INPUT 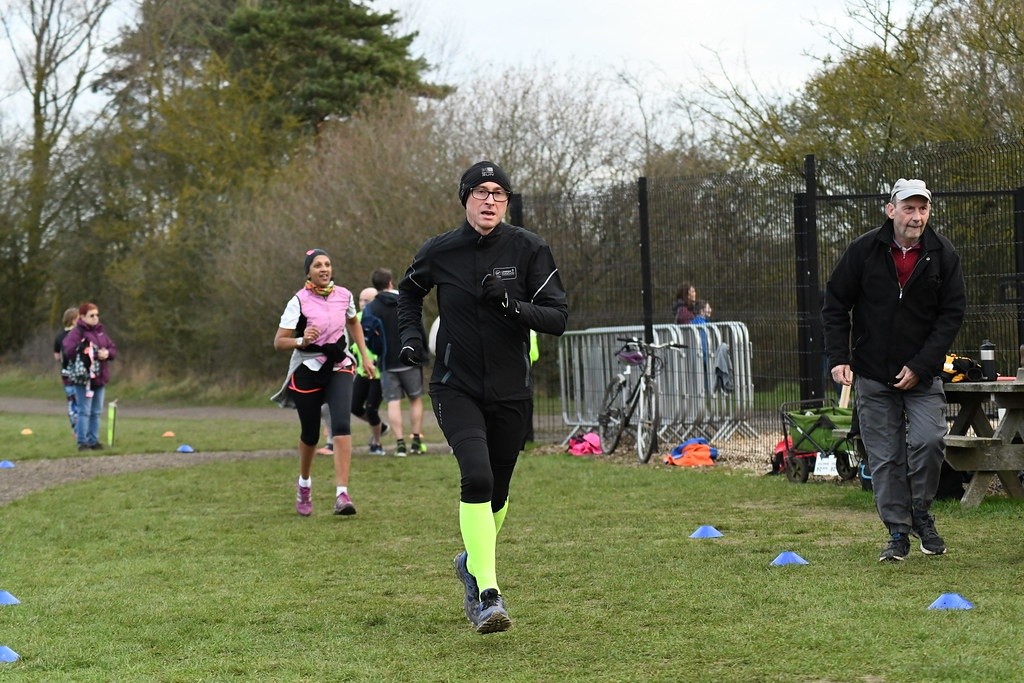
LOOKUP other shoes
[78,445,90,452]
[91,443,103,450]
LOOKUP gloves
[481,273,513,318]
[396,337,431,367]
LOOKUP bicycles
[597,336,688,463]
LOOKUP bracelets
[297,338,303,347]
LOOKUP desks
[944,378,1024,510]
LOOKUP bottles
[980,339,997,381]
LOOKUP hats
[890,177,933,203]
[458,161,512,210]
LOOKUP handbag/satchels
[358,304,387,357]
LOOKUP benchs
[833,426,1002,473]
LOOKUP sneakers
[296,482,312,517]
[368,443,385,456]
[316,443,333,455]
[454,551,481,625]
[880,533,912,561]
[324,425,329,437]
[393,445,407,457]
[409,441,427,455]
[381,422,389,436]
[476,588,511,633]
[911,514,946,555]
[333,492,356,516]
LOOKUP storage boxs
[790,407,856,450]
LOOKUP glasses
[90,314,101,317]
[468,187,511,202]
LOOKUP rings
[308,337,310,338]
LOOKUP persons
[428,315,453,455]
[312,287,391,455]
[821,179,966,562]
[271,249,377,516]
[62,303,116,450]
[53,307,79,435]
[361,268,426,456]
[530,329,539,365]
[397,162,568,634]
[674,282,711,393]
